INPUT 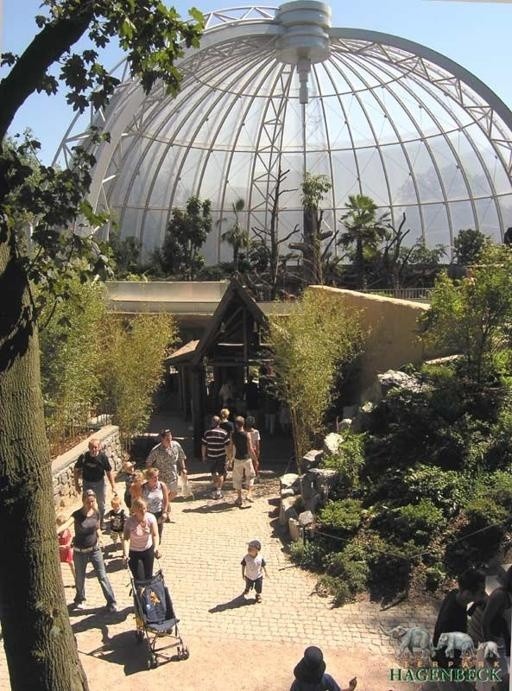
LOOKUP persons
[218,409,235,470]
[278,403,290,433]
[73,438,117,531]
[56,513,76,580]
[114,461,142,509]
[121,498,162,585]
[140,467,170,546]
[241,540,267,603]
[228,415,261,504]
[243,414,262,480]
[262,393,278,434]
[56,488,117,613]
[290,645,358,690]
[201,414,231,500]
[239,374,259,412]
[145,427,188,523]
[55,514,76,589]
[431,567,478,670]
[102,494,126,557]
[465,564,512,690]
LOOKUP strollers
[125,553,190,670]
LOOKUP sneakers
[216,493,224,498]
[256,594,262,603]
[106,602,118,612]
[246,496,254,503]
[233,496,243,505]
[210,492,217,499]
[74,600,85,609]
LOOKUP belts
[72,544,98,552]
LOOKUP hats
[246,540,261,551]
[293,646,326,684]
[81,488,96,497]
